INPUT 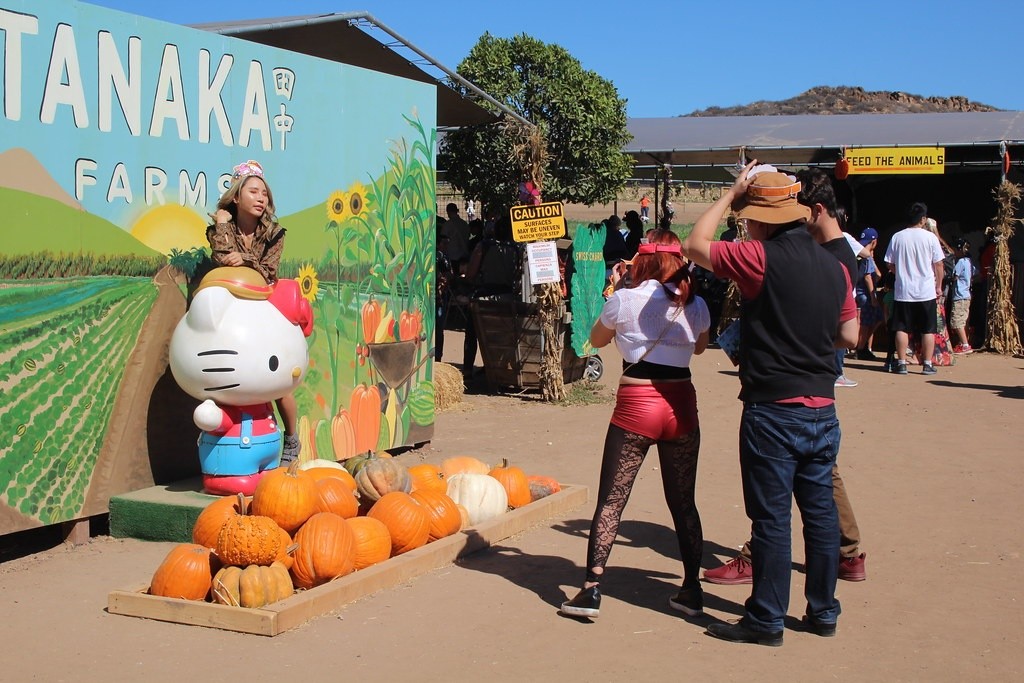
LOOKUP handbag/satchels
[448,279,476,306]
[855,284,871,308]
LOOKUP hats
[955,237,973,258]
[623,210,638,221]
[858,228,878,248]
[735,170,813,224]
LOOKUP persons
[433,194,644,378]
[560,227,718,621]
[638,194,650,224]
[207,158,304,470]
[701,182,869,584]
[677,158,850,646]
[717,197,1000,389]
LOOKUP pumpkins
[275,527,298,571]
[191,491,253,548]
[365,492,431,556]
[446,473,508,526]
[345,517,391,571]
[316,477,358,519]
[456,504,469,531]
[252,458,319,532]
[217,514,280,567]
[441,456,490,476]
[345,449,393,479]
[408,464,447,494]
[410,488,462,544]
[355,457,412,502]
[150,542,216,603]
[305,467,361,500]
[489,458,531,509]
[526,475,560,501]
[289,512,356,590]
[301,460,350,474]
[210,562,293,610]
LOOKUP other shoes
[707,334,720,345]
[463,367,485,384]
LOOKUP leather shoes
[707,617,784,647]
[802,615,837,637]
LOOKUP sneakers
[560,585,601,617]
[669,586,704,616]
[843,353,858,359]
[702,556,753,585]
[857,348,876,360]
[832,375,856,387]
[279,432,301,467]
[953,343,973,354]
[881,361,895,373]
[894,361,908,374]
[920,364,937,375]
[802,551,866,581]
